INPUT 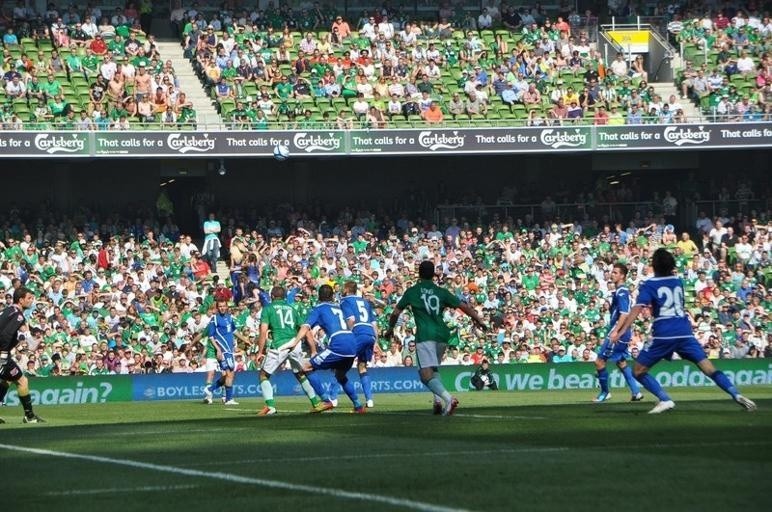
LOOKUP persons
[593,249,759,415]
[257,282,379,417]
[1,181,772,377]
[0,287,46,425]
[386,261,488,416]
[470,359,497,390]
[1,1,772,131]
[183,298,254,405]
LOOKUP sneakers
[366,399,375,408]
[433,399,442,415]
[0,416,6,425]
[732,391,758,413]
[22,414,47,425]
[646,399,677,415]
[630,392,644,402]
[591,391,613,404]
[255,405,278,417]
[329,398,338,407]
[351,406,368,415]
[202,385,213,405]
[442,396,459,417]
[224,399,240,406]
[311,400,334,413]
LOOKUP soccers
[272,144,289,160]
